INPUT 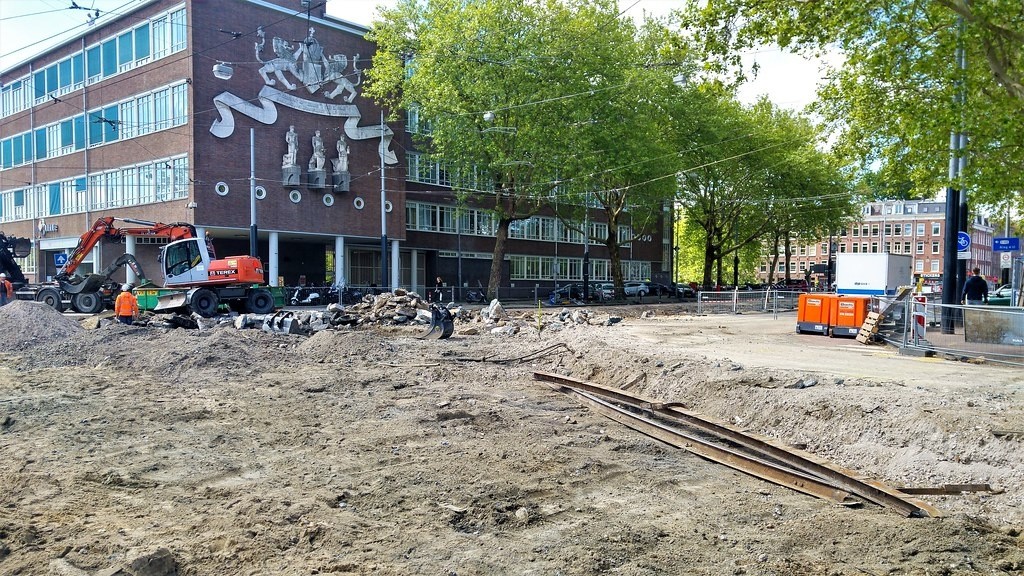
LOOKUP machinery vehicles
[0,235,161,314]
[53,216,276,318]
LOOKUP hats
[0,273,6,278]
[121,284,130,290]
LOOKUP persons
[0,273,13,306]
[961,267,989,304]
[434,277,443,299]
[115,285,138,325]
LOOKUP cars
[595,283,614,302]
[554,283,595,302]
[670,282,696,298]
[623,282,649,297]
[697,278,809,293]
[982,284,1011,306]
[622,280,669,297]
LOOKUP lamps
[213,63,233,80]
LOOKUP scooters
[465,279,487,303]
